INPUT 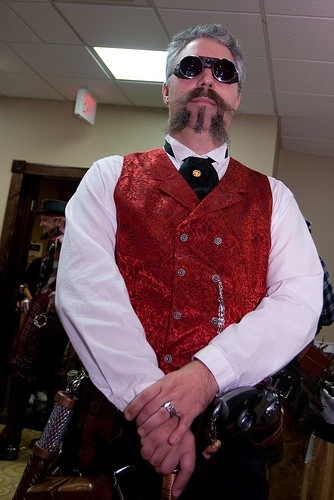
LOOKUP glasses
[167,55,241,89]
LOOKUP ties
[164,140,228,202]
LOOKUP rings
[164,402,176,417]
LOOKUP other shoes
[0,439,20,461]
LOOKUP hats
[35,199,66,214]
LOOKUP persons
[0,24,325,500]
